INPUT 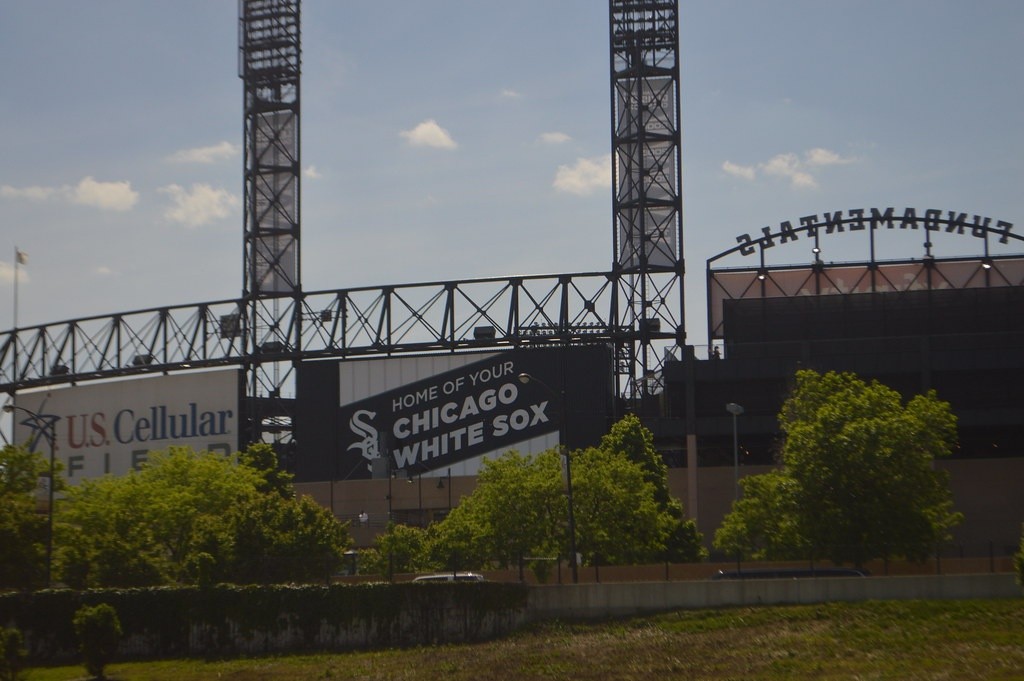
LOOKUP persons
[358,510,368,528]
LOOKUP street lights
[518,373,579,585]
[406,462,423,531]
[386,454,398,581]
[725,402,745,501]
[1,404,57,589]
[436,468,457,580]
